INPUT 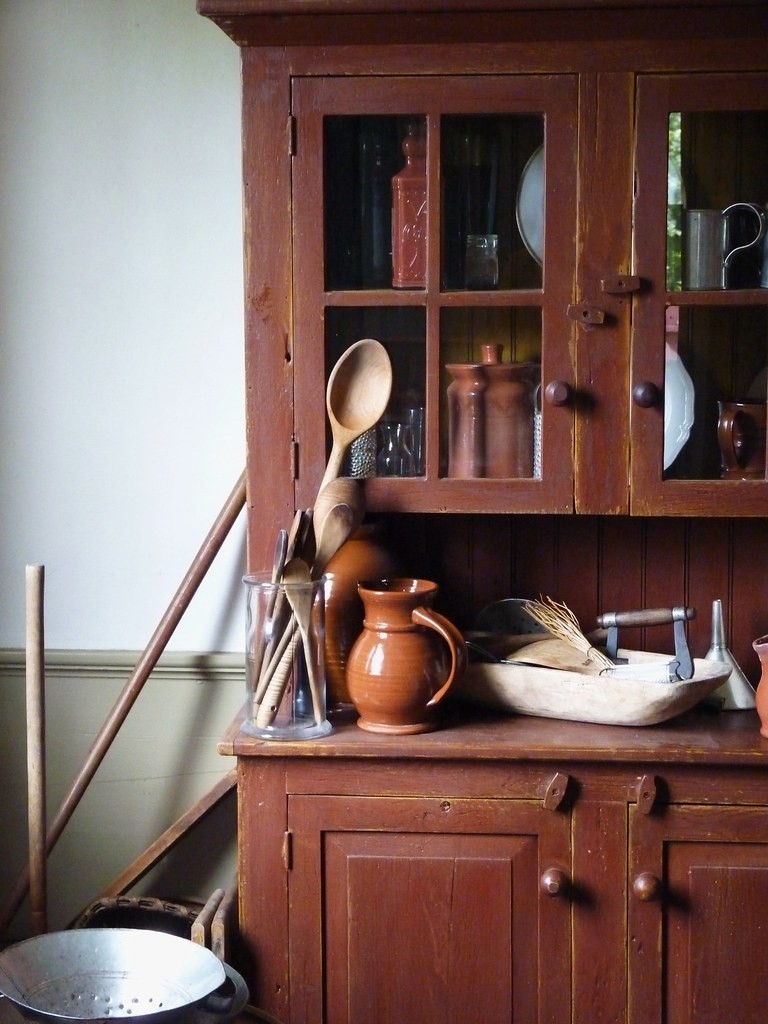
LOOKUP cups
[713,398,767,481]
[683,202,768,290]
[401,407,425,476]
[242,571,333,741]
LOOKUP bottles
[481,365,533,479]
[356,144,399,274]
[463,234,499,290]
[752,634,768,738]
[391,123,426,288]
[477,342,510,388]
[376,424,414,478]
[310,524,397,709]
[446,363,482,479]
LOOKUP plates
[514,143,544,271]
[665,340,695,472]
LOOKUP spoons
[251,338,392,727]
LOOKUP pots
[0,928,226,1024]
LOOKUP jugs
[345,577,469,736]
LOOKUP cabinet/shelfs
[196,0,768,1024]
